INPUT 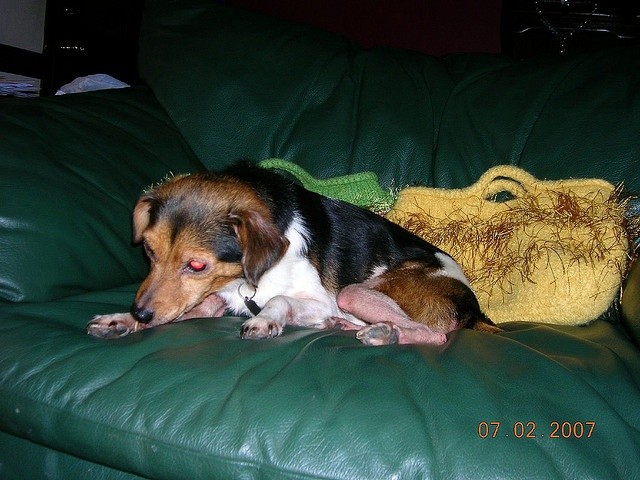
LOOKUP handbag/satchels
[257,158,396,218]
[383,165,630,326]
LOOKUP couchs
[0,0,640,478]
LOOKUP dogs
[86,155,506,346]
